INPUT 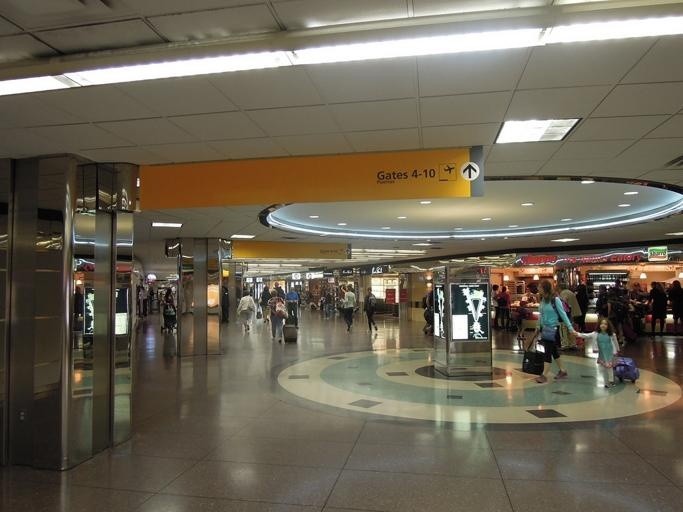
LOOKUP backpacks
[551,293,572,323]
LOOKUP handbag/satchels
[165,309,175,315]
[276,302,289,319]
[542,326,555,342]
[256,312,262,319]
[521,352,545,374]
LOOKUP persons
[160,287,174,306]
[74,286,84,318]
[422,290,434,336]
[491,278,683,389]
[364,288,378,332]
[237,282,357,344]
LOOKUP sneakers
[537,376,548,383]
[554,369,568,379]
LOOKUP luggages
[283,324,297,344]
[613,356,639,383]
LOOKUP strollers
[158,303,177,334]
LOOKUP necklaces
[461,288,486,338]
[436,288,445,338]
